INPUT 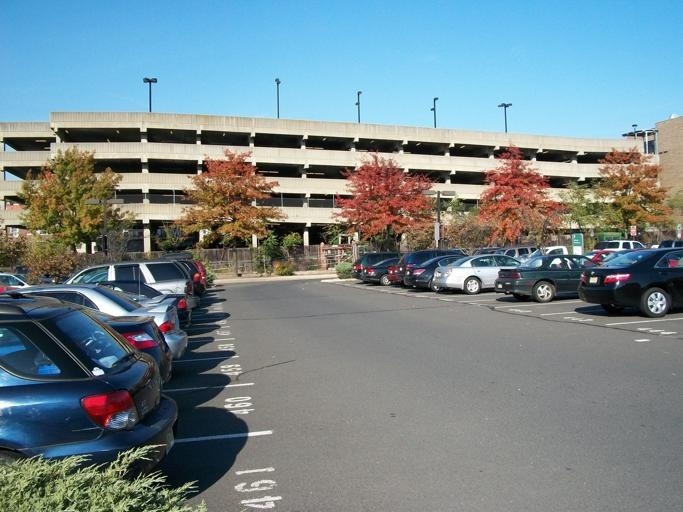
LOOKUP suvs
[1,261,207,460]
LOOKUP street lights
[356,91,363,123]
[143,77,158,114]
[430,95,441,130]
[497,102,514,132]
[275,78,284,119]
